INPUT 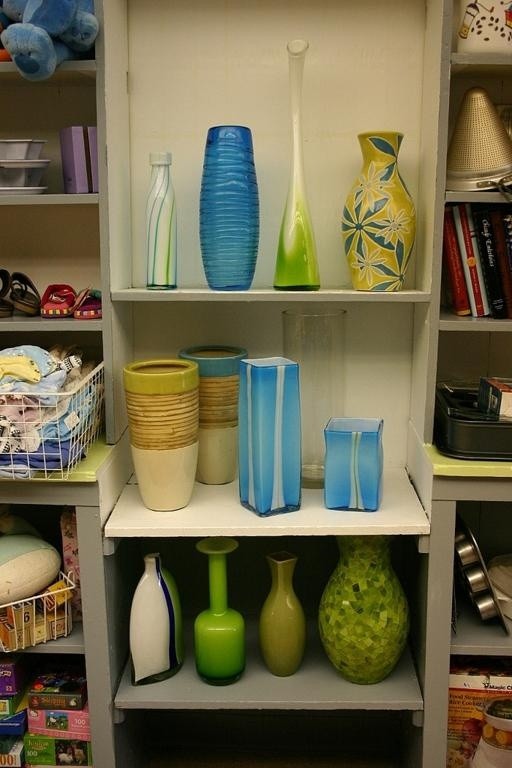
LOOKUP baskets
[0,357,105,484]
[0,569,78,654]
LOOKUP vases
[238,356,301,519]
[342,132,416,293]
[147,153,176,290]
[258,553,308,677]
[194,538,246,684]
[129,553,183,686]
[318,536,411,686]
[200,127,259,289]
[322,418,384,513]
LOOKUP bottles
[145,150,180,290]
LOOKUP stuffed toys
[1,0,101,83]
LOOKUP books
[444,203,511,321]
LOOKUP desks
[0,438,131,768]
[407,443,511,768]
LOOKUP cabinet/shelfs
[105,291,429,710]
[409,2,512,445]
[1,6,131,447]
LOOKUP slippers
[10,270,42,316]
[74,287,103,320]
[40,283,77,318]
[0,268,14,319]
